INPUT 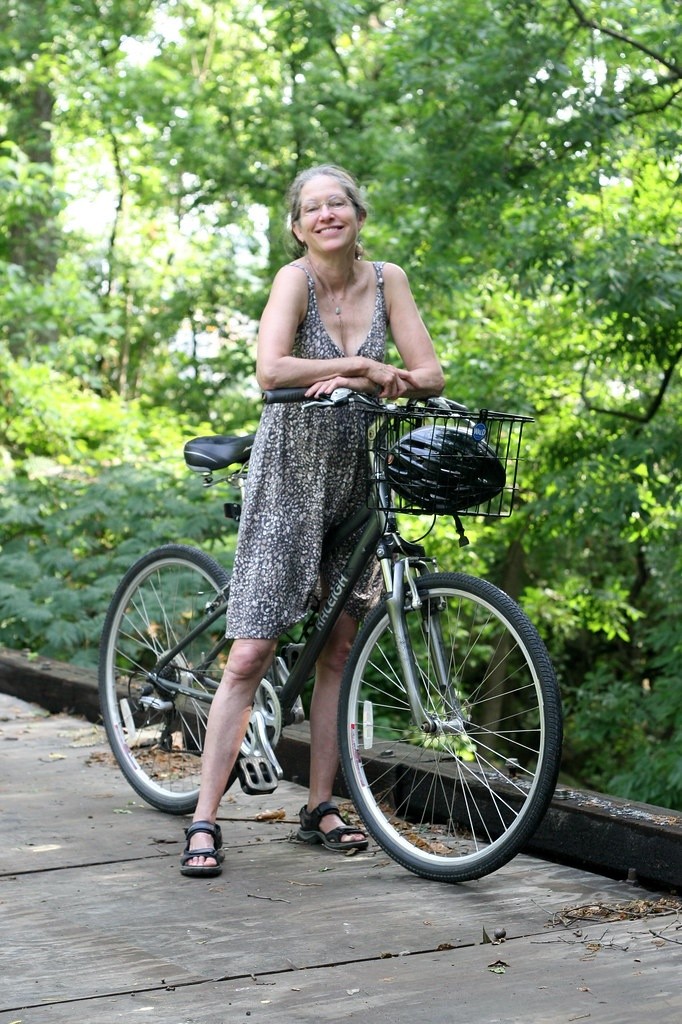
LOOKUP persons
[181,164,449,877]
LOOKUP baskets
[354,405,536,517]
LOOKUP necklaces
[306,253,355,315]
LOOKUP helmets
[385,425,506,514]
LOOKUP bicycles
[95,381,565,883]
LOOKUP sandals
[179,821,225,875]
[294,800,369,850]
